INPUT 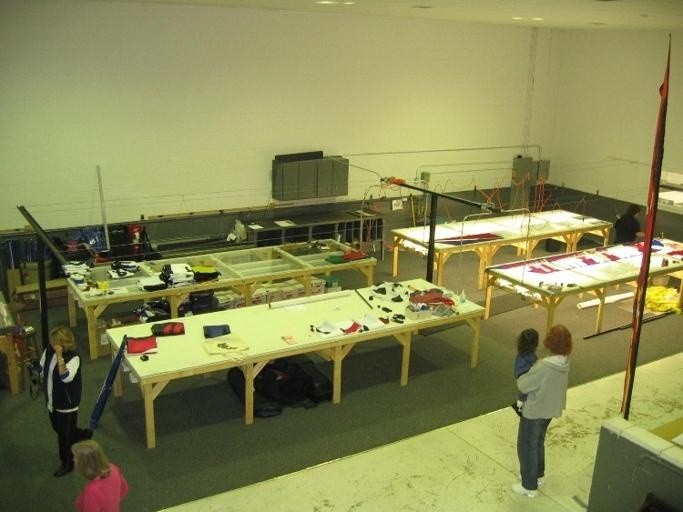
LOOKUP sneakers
[518,475,544,489]
[511,484,537,498]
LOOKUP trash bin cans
[191,291,213,314]
[545,238,561,252]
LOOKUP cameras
[25,357,44,377]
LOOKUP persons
[34,325,92,477]
[614,204,646,243]
[510,322,574,499]
[511,327,539,416]
[71,440,129,512]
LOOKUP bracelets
[57,358,65,362]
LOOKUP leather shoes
[54,464,73,477]
[511,402,521,417]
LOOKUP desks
[389,207,683,340]
